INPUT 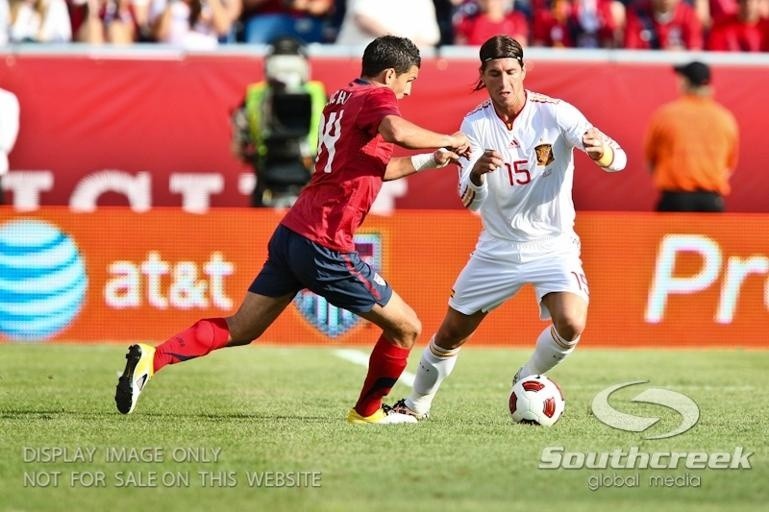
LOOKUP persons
[2,0,768,57]
[381,35,631,426]
[113,34,474,425]
[643,62,743,211]
[229,37,331,207]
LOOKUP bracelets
[410,153,435,174]
[594,144,613,168]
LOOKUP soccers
[510,375,564,426]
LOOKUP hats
[672,60,712,86]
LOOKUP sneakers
[347,398,429,427]
[114,342,158,417]
